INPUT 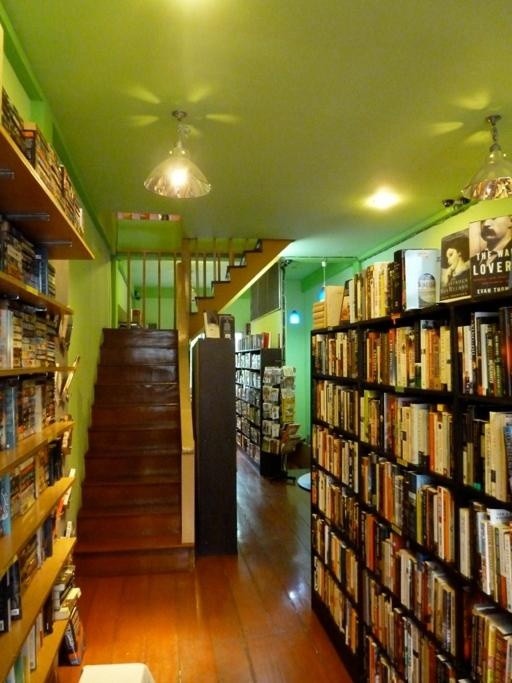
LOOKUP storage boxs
[293,443,311,468]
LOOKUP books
[235,332,302,465]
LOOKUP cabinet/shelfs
[0,113,94,683]
[236,347,296,484]
[310,290,512,682]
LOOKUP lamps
[141,110,211,200]
[460,112,511,202]
